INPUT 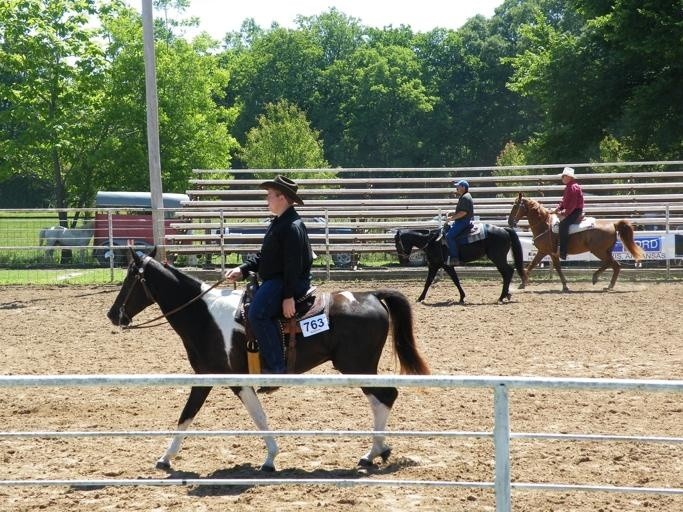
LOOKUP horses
[508,191,644,293]
[39,218,96,263]
[394,224,529,304]
[107,244,431,473]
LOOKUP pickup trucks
[211,215,365,268]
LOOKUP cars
[385,215,457,267]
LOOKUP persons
[442,179,476,266]
[215,168,316,396]
[549,167,585,260]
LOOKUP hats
[260,175,304,205]
[454,180,469,189]
[558,167,577,180]
